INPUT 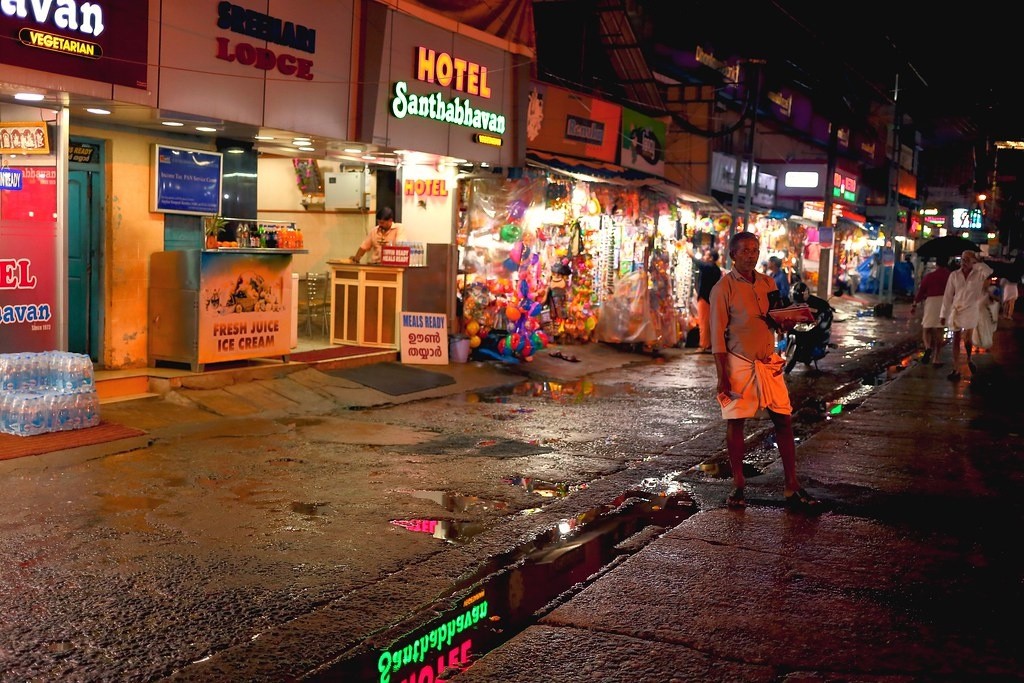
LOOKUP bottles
[243,223,249,247]
[278,226,304,249]
[258,224,278,248]
[236,222,243,247]
[0,350,99,436]
[389,240,423,267]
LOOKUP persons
[349,206,403,264]
[980,253,1024,320]
[939,250,994,380]
[680,237,722,354]
[709,232,825,508]
[761,262,770,277]
[767,256,791,357]
[911,254,954,366]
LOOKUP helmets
[793,283,809,302]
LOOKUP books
[769,306,815,325]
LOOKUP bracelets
[912,304,917,308]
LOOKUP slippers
[786,488,824,513]
[726,487,746,510]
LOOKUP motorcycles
[783,274,844,374]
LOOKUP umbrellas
[916,236,983,269]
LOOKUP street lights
[977,189,987,257]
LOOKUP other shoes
[922,348,932,364]
[947,369,960,381]
[968,361,976,373]
